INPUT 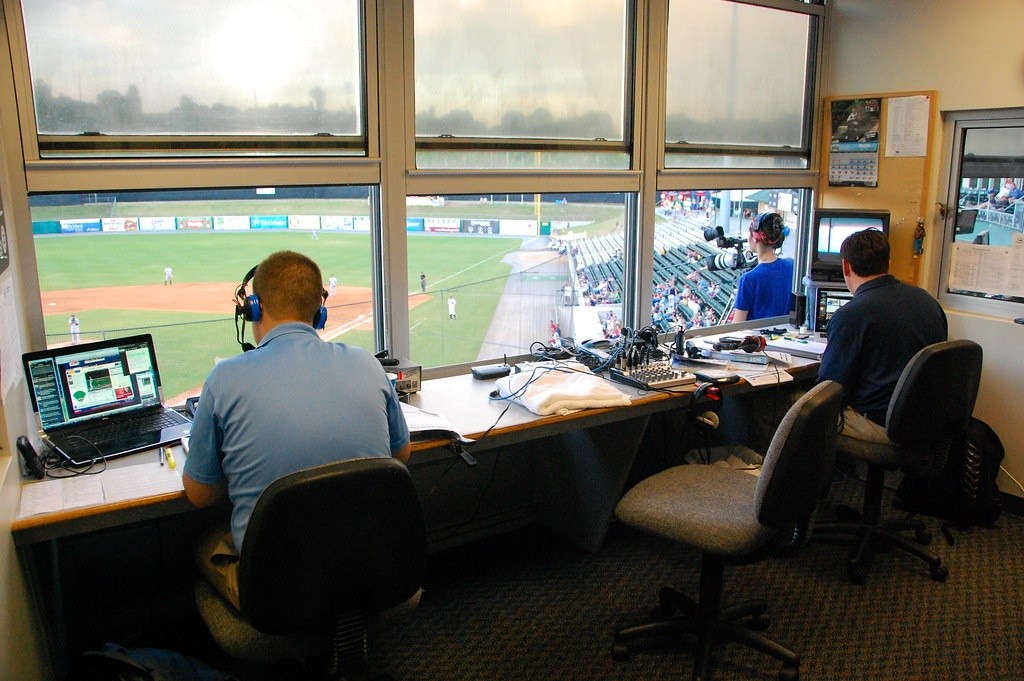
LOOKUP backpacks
[893,416,1004,528]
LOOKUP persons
[312,228,319,240]
[448,296,457,320]
[845,109,860,139]
[163,264,173,286]
[420,271,426,292]
[548,189,756,342]
[968,178,1023,213]
[729,211,794,324]
[774,227,947,462]
[67,313,80,345]
[181,250,412,613]
[329,274,337,299]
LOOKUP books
[766,336,828,360]
[710,351,773,364]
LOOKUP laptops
[812,286,854,344]
[21,333,195,469]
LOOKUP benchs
[568,204,747,332]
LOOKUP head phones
[713,336,767,353]
[753,213,785,248]
[238,264,329,330]
[683,382,725,433]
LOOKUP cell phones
[16,435,46,480]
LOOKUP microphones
[236,305,256,352]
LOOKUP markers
[166,448,176,468]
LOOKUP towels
[495,369,633,416]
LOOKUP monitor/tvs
[972,231,989,245]
[808,207,890,282]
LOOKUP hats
[988,190,996,195]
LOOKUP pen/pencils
[784,337,808,343]
[159,447,164,465]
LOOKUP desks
[12,326,830,681]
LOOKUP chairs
[805,341,982,584]
[612,381,842,681]
[218,458,427,681]
